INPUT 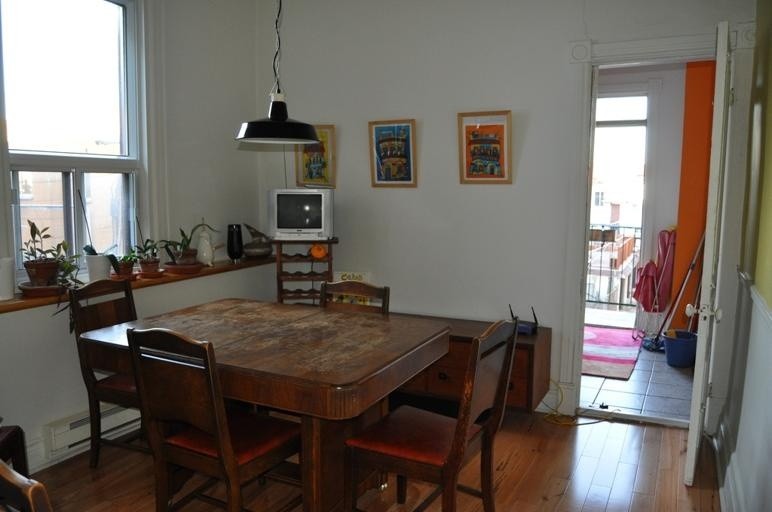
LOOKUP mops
[641,231,705,352]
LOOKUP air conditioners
[42,403,141,468]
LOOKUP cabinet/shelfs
[389,312,553,414]
[266,235,339,305]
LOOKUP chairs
[319,279,390,313]
[67,277,142,470]
[343,320,521,511]
[126,327,302,511]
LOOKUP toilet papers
[0,257,16,301]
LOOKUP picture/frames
[367,118,417,188]
[456,109,513,186]
[294,123,336,189]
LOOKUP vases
[196,229,217,267]
[243,224,275,259]
[227,224,245,265]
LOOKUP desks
[77,296,451,512]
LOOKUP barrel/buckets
[662,330,698,368]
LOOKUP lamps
[233,0,321,146]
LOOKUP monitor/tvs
[268,189,333,241]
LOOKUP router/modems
[509,303,538,336]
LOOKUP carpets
[580,326,646,381]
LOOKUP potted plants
[134,238,171,278]
[84,243,120,282]
[18,218,86,334]
[115,247,143,276]
[161,223,223,274]
